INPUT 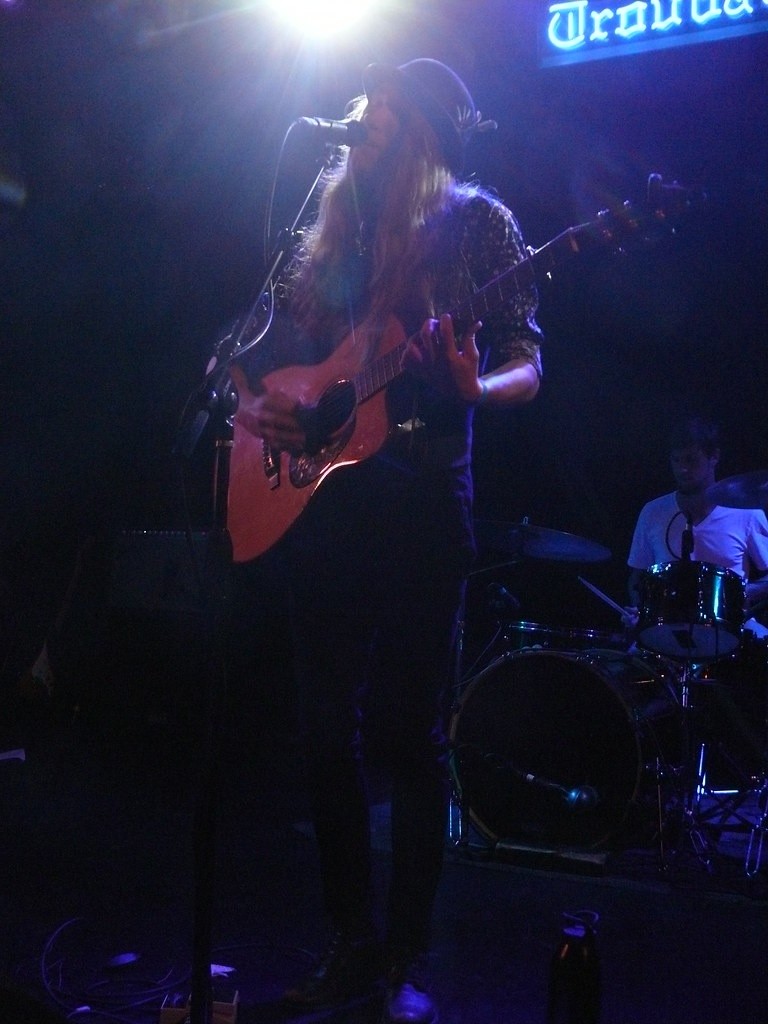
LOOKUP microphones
[523,774,598,814]
[300,117,369,147]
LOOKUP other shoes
[387,974,441,1024]
[274,959,374,1024]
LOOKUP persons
[206,59,542,1023]
[629,427,767,646]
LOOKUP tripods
[633,617,768,881]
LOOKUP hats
[652,416,718,459]
[363,56,481,175]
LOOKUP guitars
[205,165,708,571]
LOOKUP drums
[503,612,627,650]
[444,641,687,862]
[628,558,749,664]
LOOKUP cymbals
[464,514,615,564]
[704,466,768,510]
